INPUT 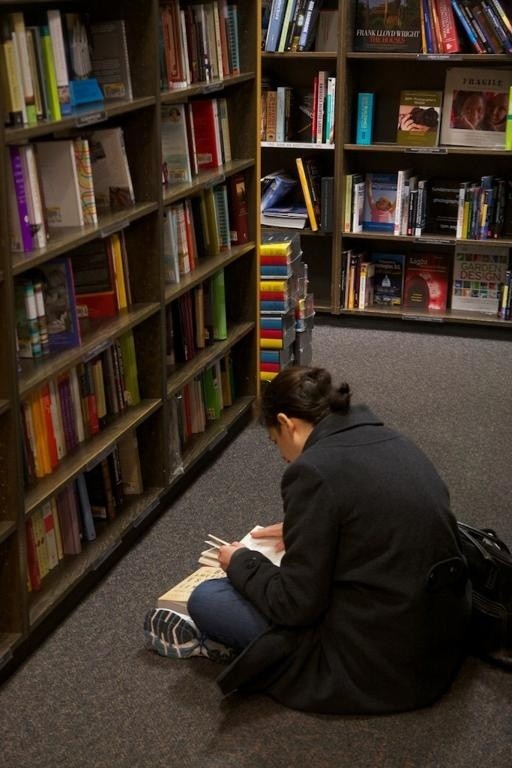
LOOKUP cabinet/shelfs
[262,0,510,330]
[1,0,258,670]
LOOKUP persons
[144,365,473,717]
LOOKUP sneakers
[142,606,234,664]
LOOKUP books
[3,1,285,615]
[257,1,512,381]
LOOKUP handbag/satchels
[455,520,512,672]
